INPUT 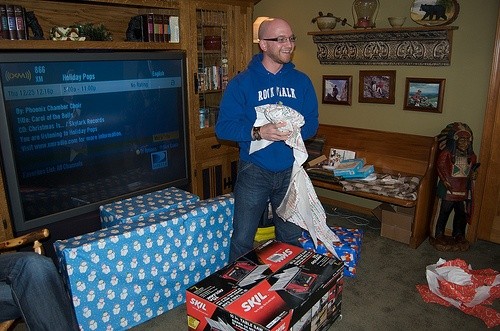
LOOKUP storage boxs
[343,164,376,179]
[370,202,415,248]
[329,146,355,167]
[52,185,239,331]
[184,238,346,331]
[334,158,366,177]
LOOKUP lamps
[252,16,269,53]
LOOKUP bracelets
[253,127,262,140]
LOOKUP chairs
[0,227,52,331]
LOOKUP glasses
[264,36,296,43]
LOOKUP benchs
[298,126,442,251]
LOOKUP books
[134,13,179,43]
[0,4,44,40]
[199,105,219,129]
[199,65,221,92]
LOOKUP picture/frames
[358,69,397,105]
[403,76,447,114]
[321,74,353,106]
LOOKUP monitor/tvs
[0,50,193,235]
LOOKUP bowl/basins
[317,17,336,32]
[387,17,406,27]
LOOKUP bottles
[203,36,220,50]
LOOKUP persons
[0,252,80,331]
[371,77,384,93]
[412,89,423,106]
[333,85,339,99]
[437,121,480,244]
[216,18,319,262]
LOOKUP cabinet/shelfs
[0,0,259,252]
